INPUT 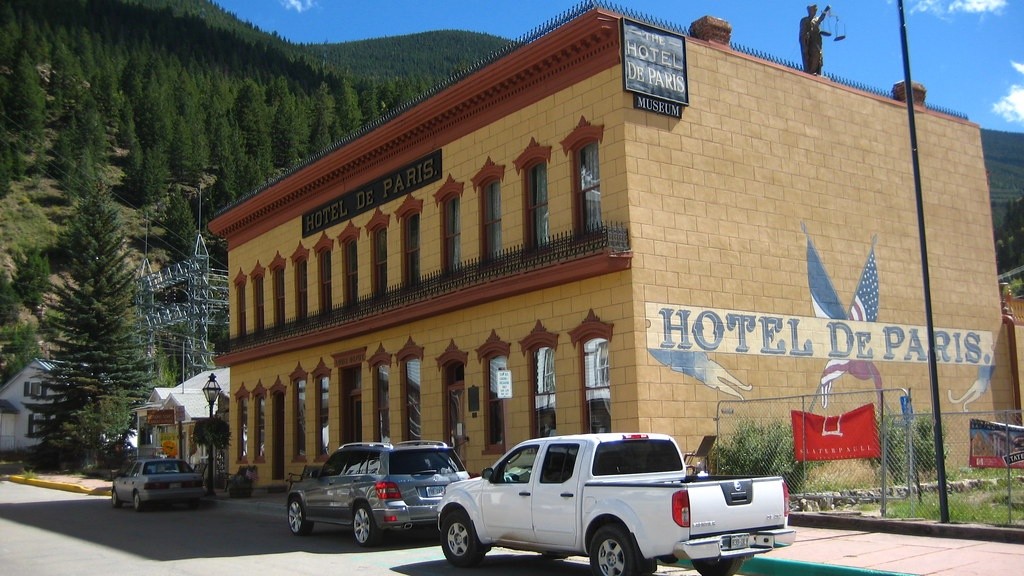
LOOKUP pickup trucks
[437,433,796,576]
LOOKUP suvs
[287,440,472,547]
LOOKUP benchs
[286,464,323,490]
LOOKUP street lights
[202,373,222,496]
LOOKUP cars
[111,460,203,512]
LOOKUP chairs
[144,469,152,474]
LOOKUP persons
[799,3,830,75]
